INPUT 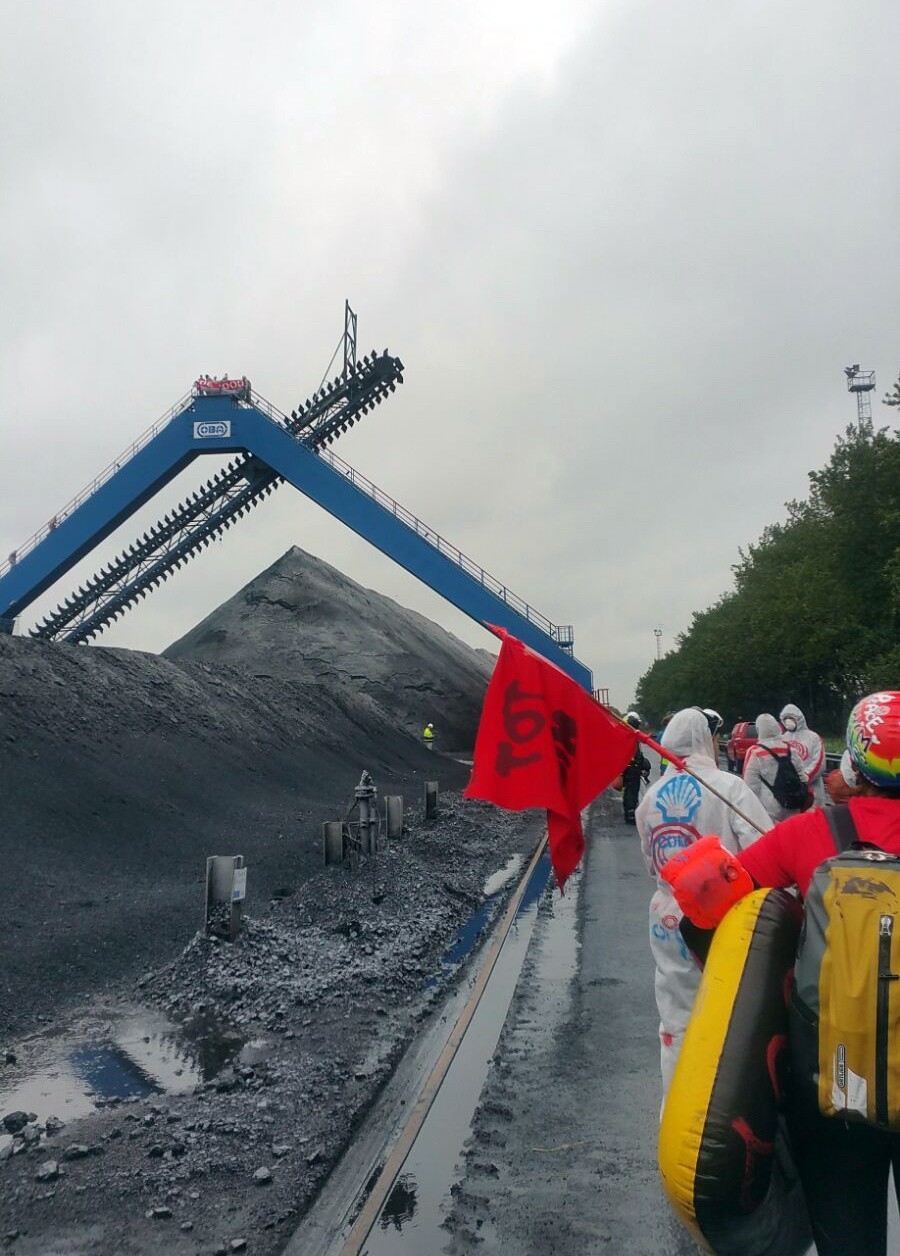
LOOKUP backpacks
[754,741,809,810]
[791,792,900,1136]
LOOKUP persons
[198,372,228,386]
[779,705,826,808]
[8,551,16,566]
[424,724,434,749]
[657,708,719,775]
[48,518,56,530]
[658,692,900,1256]
[743,714,810,826]
[636,709,774,1120]
[614,712,651,825]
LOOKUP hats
[428,723,434,729]
[696,707,721,741]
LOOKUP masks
[783,718,798,731]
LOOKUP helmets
[842,690,900,791]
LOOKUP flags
[463,628,636,893]
